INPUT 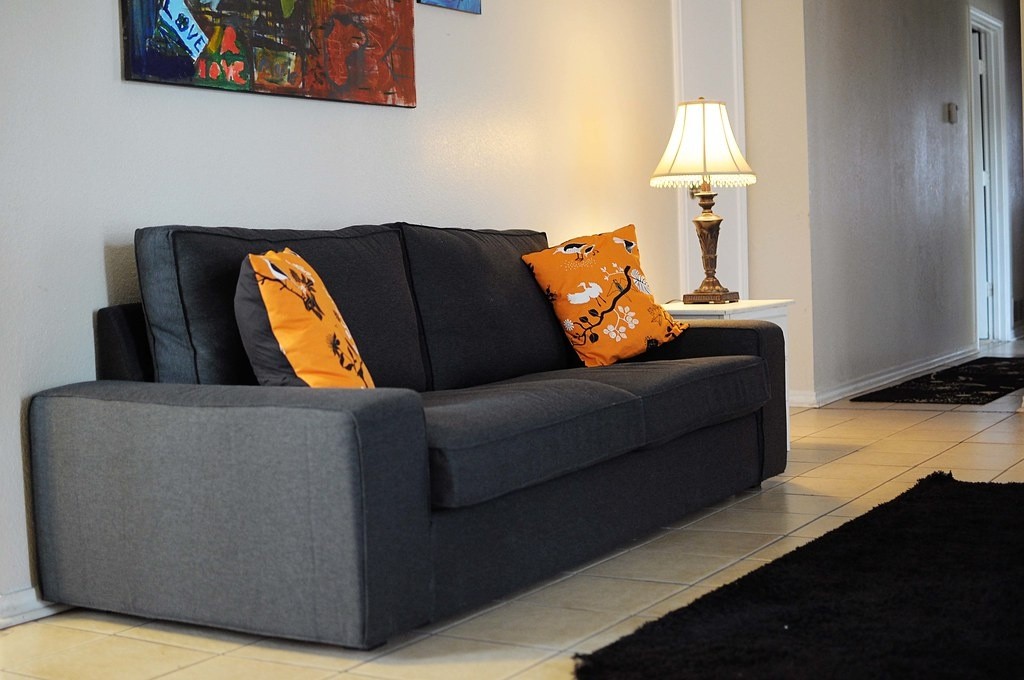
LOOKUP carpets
[571,470,1024,680]
[850,357,1024,405]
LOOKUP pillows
[522,224,690,368]
[233,246,377,389]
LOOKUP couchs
[30,221,786,653]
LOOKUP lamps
[650,95,756,304]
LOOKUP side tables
[663,299,798,451]
